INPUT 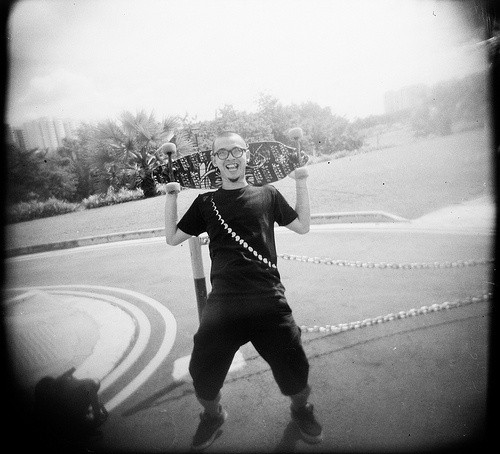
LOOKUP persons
[165,131,326,446]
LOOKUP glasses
[212,147,246,160]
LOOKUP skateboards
[152,128,308,194]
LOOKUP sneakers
[290,402,323,442]
[192,404,228,450]
